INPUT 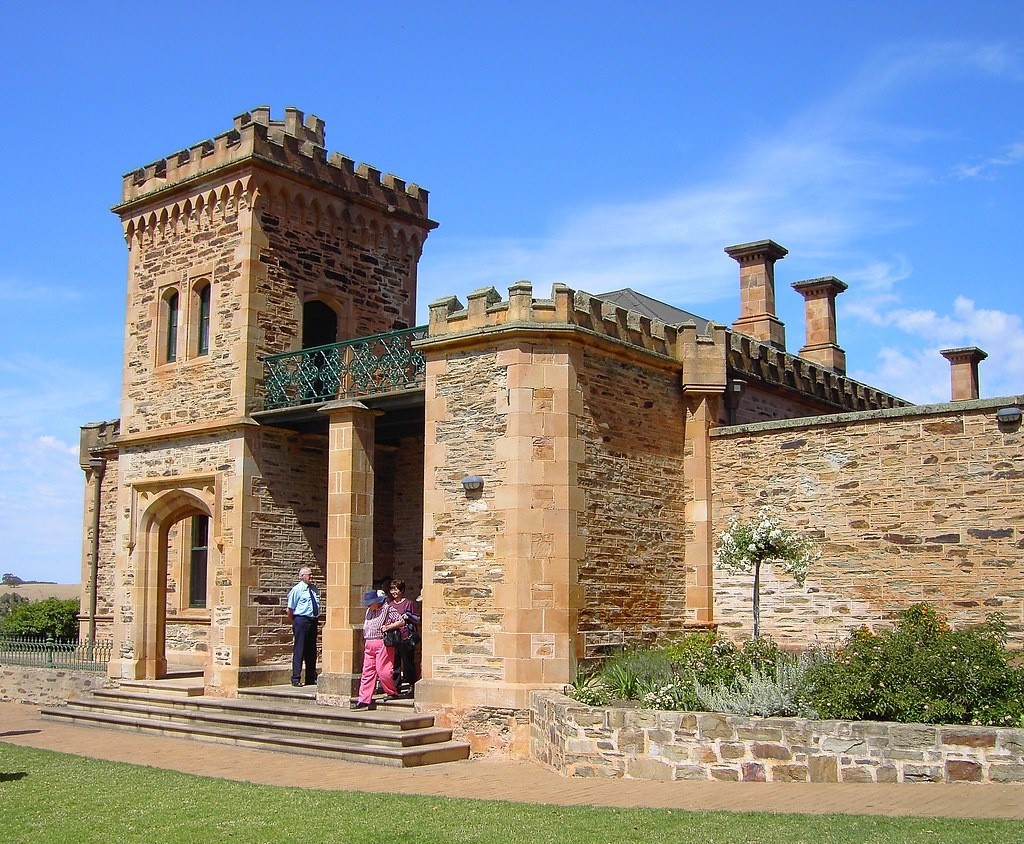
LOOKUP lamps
[461,476,484,492]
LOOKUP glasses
[302,573,314,576]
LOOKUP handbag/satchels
[407,631,421,647]
[383,627,403,647]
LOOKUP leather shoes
[292,681,303,687]
[306,681,317,685]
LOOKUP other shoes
[350,701,369,709]
[383,694,398,700]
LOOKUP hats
[360,590,384,607]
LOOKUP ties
[308,585,319,616]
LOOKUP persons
[285,567,320,686]
[350,589,405,711]
[386,579,422,700]
